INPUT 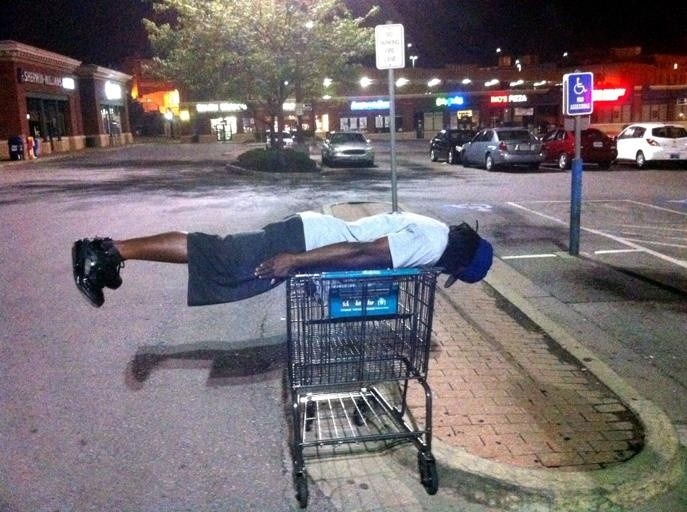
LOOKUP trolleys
[279,266,442,511]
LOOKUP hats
[444,238,493,288]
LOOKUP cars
[320,129,375,168]
[428,118,687,171]
[262,128,296,150]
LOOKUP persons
[162,107,175,139]
[70,209,493,309]
[7,134,39,161]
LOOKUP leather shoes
[94,233,124,290]
[71,237,104,309]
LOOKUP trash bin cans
[7,136,25,161]
[85,137,95,147]
